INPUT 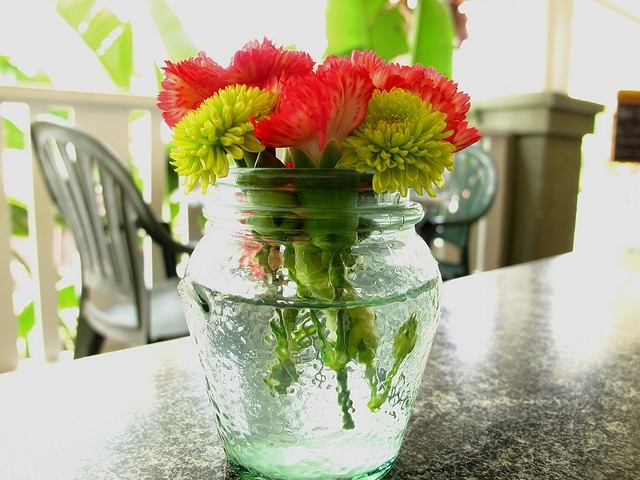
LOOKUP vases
[178,166,444,480]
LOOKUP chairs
[31,120,194,361]
[415,144,499,281]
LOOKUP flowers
[154,37,485,198]
